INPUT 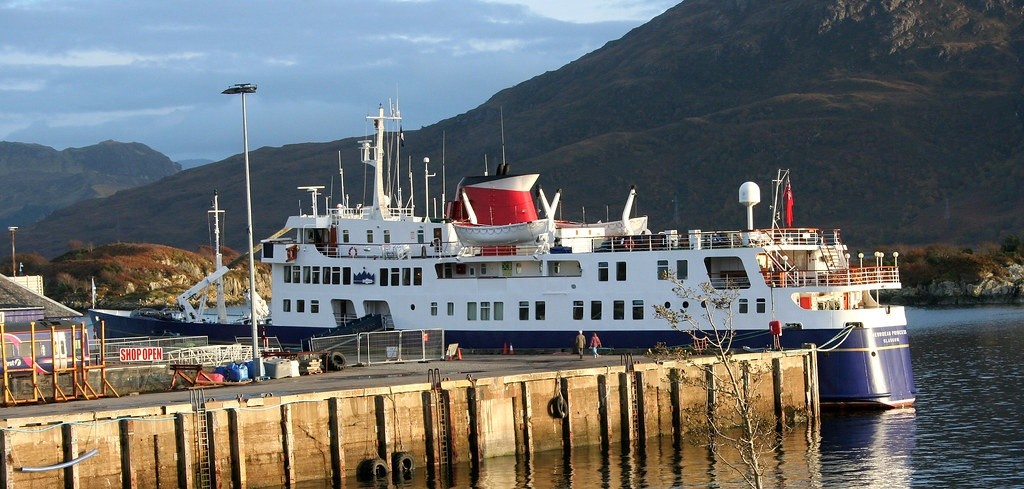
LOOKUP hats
[579,330,582,333]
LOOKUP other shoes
[594,354,599,358]
[579,355,583,359]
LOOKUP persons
[589,332,601,359]
[576,329,586,359]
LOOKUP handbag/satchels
[589,346,592,349]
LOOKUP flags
[400,123,405,147]
[784,181,793,227]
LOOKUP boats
[85,93,919,413]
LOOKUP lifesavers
[394,453,415,471]
[348,247,358,258]
[625,239,635,251]
[329,352,347,371]
[553,397,569,418]
[358,460,389,477]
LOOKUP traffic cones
[448,350,453,361]
[502,342,509,354]
[508,342,515,355]
[457,348,462,360]
